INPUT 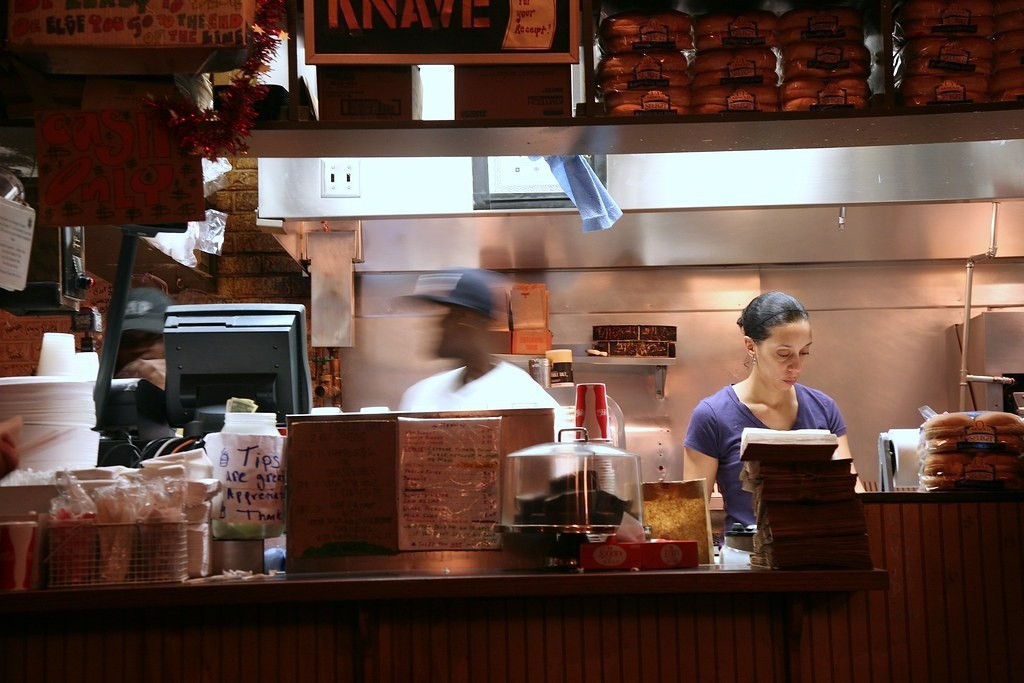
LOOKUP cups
[38,331,77,376]
[528,358,552,389]
[0,522,37,589]
[574,383,616,497]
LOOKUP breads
[596,0,1024,117]
[920,411,1024,491]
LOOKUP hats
[121,287,171,335]
[413,268,508,321]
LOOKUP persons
[683,291,865,570]
[398,269,573,442]
[112,287,176,390]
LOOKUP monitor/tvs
[164,304,312,438]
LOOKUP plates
[0,375,101,469]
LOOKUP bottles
[220,413,283,437]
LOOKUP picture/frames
[471,154,608,209]
